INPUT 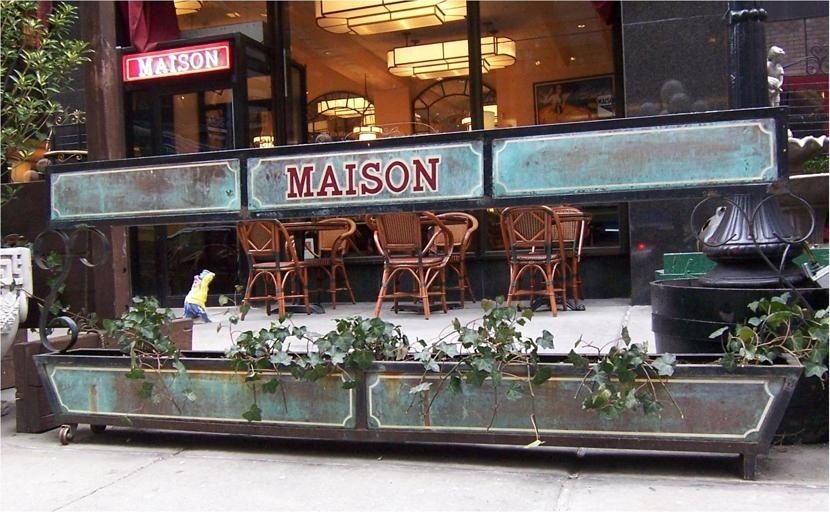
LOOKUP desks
[517,211,591,312]
[270,221,347,314]
[390,214,467,314]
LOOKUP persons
[768,45,787,107]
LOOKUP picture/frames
[531,71,617,123]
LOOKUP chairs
[531,205,587,299]
[500,205,568,317]
[236,219,309,321]
[432,212,479,308]
[366,210,454,319]
[285,217,358,308]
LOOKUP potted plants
[30,299,828,481]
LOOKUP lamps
[314,0,516,118]
